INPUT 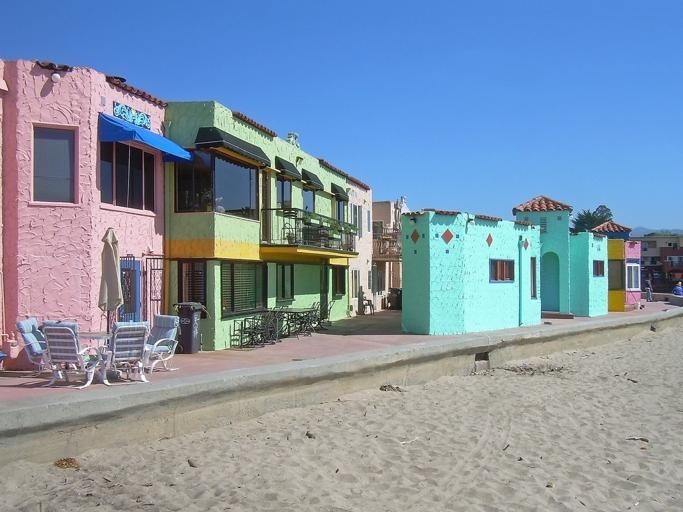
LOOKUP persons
[644,276,653,302]
[671,281,683,296]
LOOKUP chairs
[284,218,354,255]
[13,314,180,389]
[232,300,339,347]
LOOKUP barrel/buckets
[633,302,641,311]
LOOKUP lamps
[33,61,73,83]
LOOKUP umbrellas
[99,228,124,345]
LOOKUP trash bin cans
[172,302,203,354]
[389,287,402,310]
